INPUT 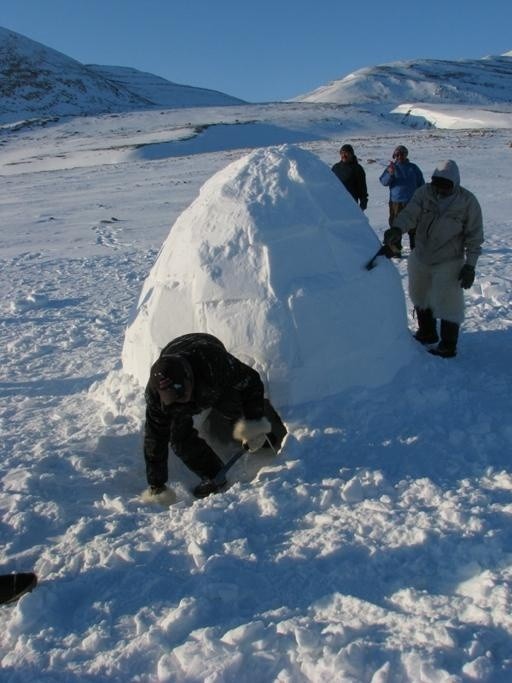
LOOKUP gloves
[232,418,272,450]
[458,264,475,289]
[384,227,402,253]
[141,484,176,504]
[360,198,367,209]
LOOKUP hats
[340,144,353,153]
[395,146,407,154]
[151,362,185,401]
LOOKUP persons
[379,145,426,259]
[142,333,288,497]
[382,158,485,358]
[331,144,369,212]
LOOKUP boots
[429,319,458,357]
[413,307,438,344]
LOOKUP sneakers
[194,469,225,498]
